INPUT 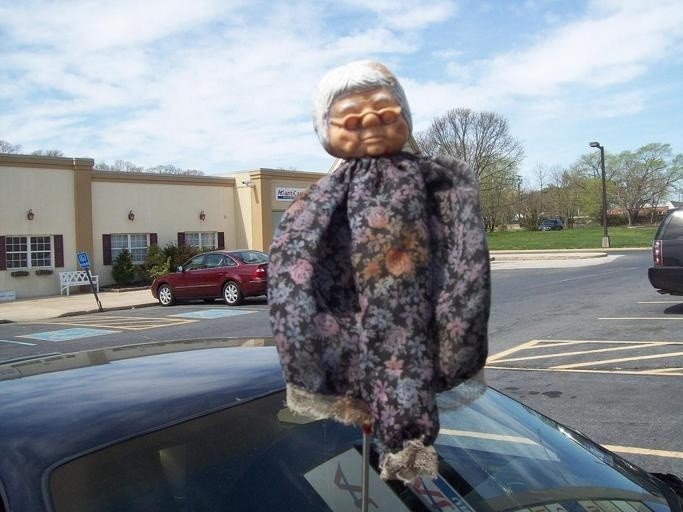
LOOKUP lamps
[199,210,205,221]
[128,209,135,221]
[28,208,34,220]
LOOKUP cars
[538,219,564,231]
[151,249,270,307]
[2,337,683,512]
[648,208,683,296]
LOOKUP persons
[268,59,491,488]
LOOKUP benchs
[57,270,100,295]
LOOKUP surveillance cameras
[243,181,249,184]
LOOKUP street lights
[589,142,610,248]
